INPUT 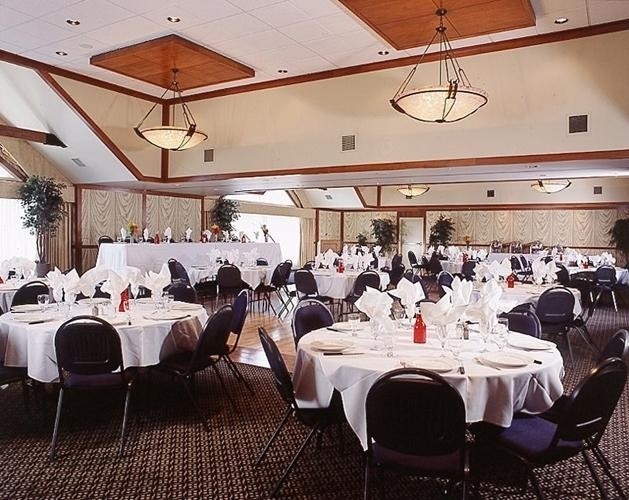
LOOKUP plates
[331,321,365,330]
[11,304,54,325]
[169,302,203,311]
[477,349,534,367]
[138,310,187,320]
[309,338,353,352]
[399,359,452,372]
[507,336,557,350]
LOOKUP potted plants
[18,176,68,277]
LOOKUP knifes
[458,359,466,375]
[327,327,347,334]
[322,352,365,356]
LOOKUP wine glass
[346,313,360,339]
[57,290,174,325]
[310,263,316,272]
[115,228,268,244]
[469,270,559,295]
[9,275,17,289]
[37,293,49,314]
[366,301,510,357]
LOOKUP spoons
[472,357,501,371]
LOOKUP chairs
[98,235,113,247]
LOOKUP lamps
[397,183,429,197]
[133,69,208,151]
[531,179,570,194]
[389,0,489,123]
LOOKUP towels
[120,227,259,243]
